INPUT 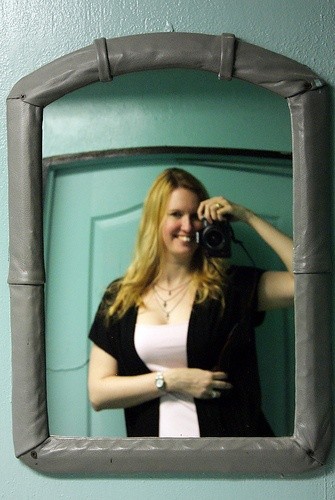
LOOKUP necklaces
[147,280,192,318]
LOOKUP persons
[88,168,294,439]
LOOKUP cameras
[196,216,233,258]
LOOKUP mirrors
[8,32,335,478]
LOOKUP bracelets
[155,371,166,391]
